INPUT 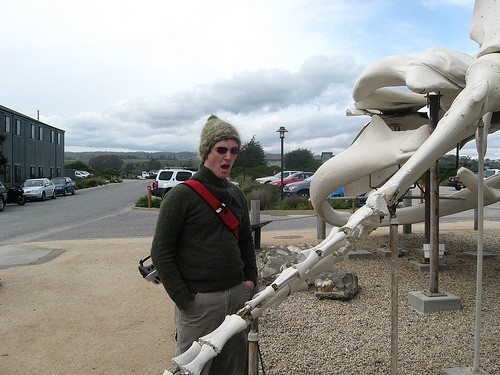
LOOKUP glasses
[212,146,239,154]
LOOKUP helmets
[138,254,161,284]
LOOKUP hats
[199,115,241,160]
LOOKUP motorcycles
[6,184,26,205]
[0,181,7,212]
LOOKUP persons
[150,115,259,375]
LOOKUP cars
[326,187,367,205]
[269,172,315,190]
[22,178,59,201]
[447,168,500,191]
[75,171,88,179]
[283,176,313,197]
[255,170,309,185]
[50,177,77,197]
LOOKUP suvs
[151,166,199,200]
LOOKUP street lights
[276,125,289,201]
